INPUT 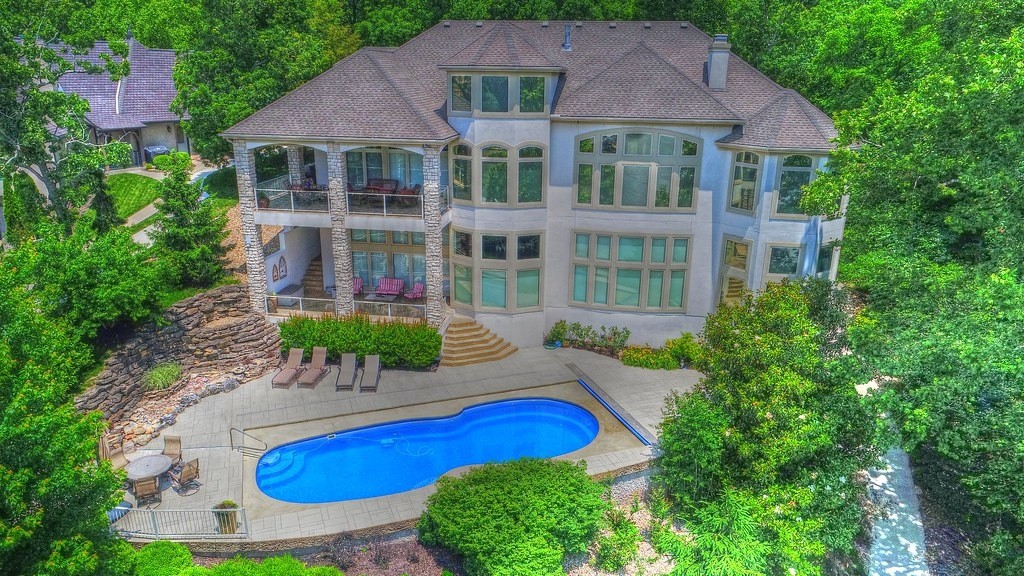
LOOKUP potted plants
[257,191,270,208]
[267,291,278,313]
[211,500,240,534]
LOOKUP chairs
[133,475,162,510]
[352,277,363,300]
[358,355,382,393]
[403,282,424,305]
[335,353,358,392]
[347,182,357,202]
[296,346,331,389]
[159,435,183,478]
[271,348,308,390]
[122,440,140,463]
[398,184,421,210]
[283,179,306,202]
[167,458,200,496]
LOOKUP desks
[124,454,172,482]
[360,196,393,208]
[364,294,399,303]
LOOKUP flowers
[543,318,618,356]
[620,337,681,370]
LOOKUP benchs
[374,277,405,299]
[360,179,399,202]
